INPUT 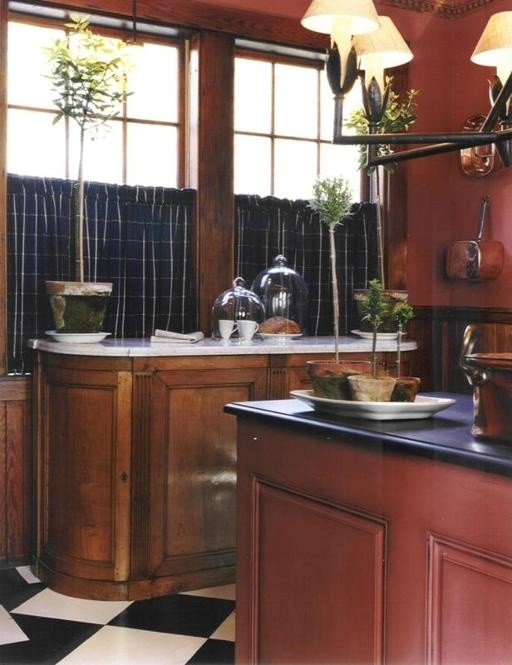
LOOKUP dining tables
[301,179,374,402]
[37,11,135,341]
[389,302,423,401]
[347,277,398,402]
[338,71,416,339]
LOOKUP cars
[236,318,261,341]
[218,319,238,340]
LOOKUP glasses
[297,0,512,172]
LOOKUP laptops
[258,316,301,334]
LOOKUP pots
[460,350,512,445]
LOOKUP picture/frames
[221,385,512,664]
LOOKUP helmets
[291,389,458,419]
[43,329,113,344]
[350,327,407,340]
[257,332,304,340]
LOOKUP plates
[18,340,427,600]
